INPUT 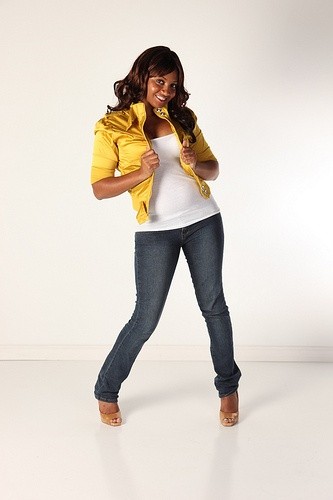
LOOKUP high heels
[219,391,239,427]
[99,408,122,427]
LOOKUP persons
[90,44,241,427]
